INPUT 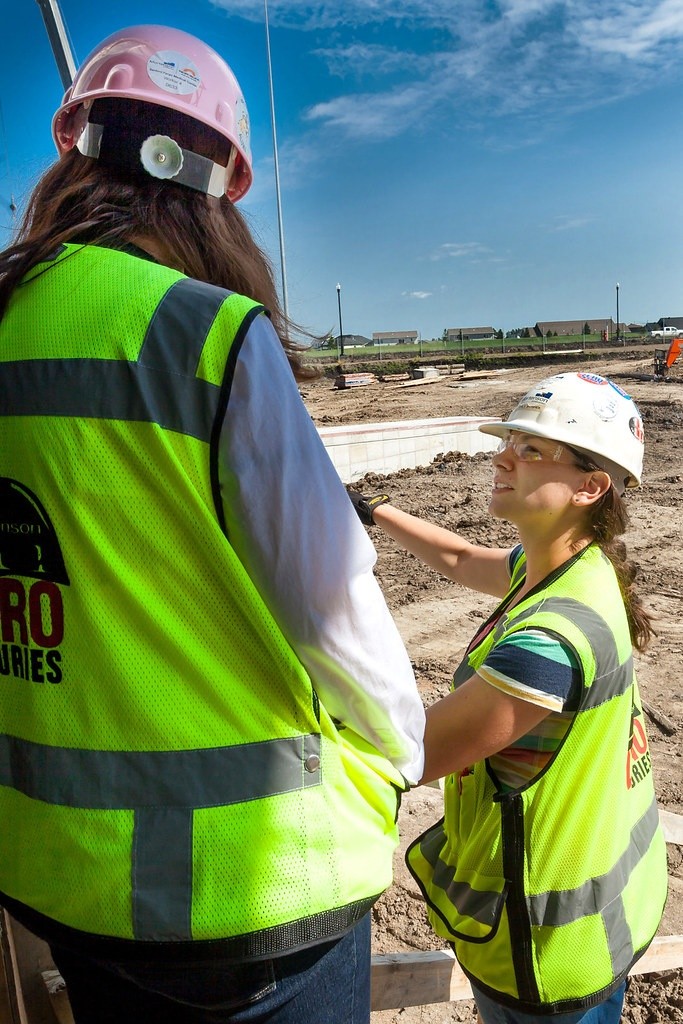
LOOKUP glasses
[497,434,592,472]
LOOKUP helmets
[477,372,643,489]
[52,26,253,204]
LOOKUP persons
[347,367,671,1024]
[0,15,421,1024]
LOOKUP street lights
[616,282,620,339]
[336,283,344,357]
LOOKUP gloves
[348,491,391,526]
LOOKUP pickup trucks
[650,326,683,339]
